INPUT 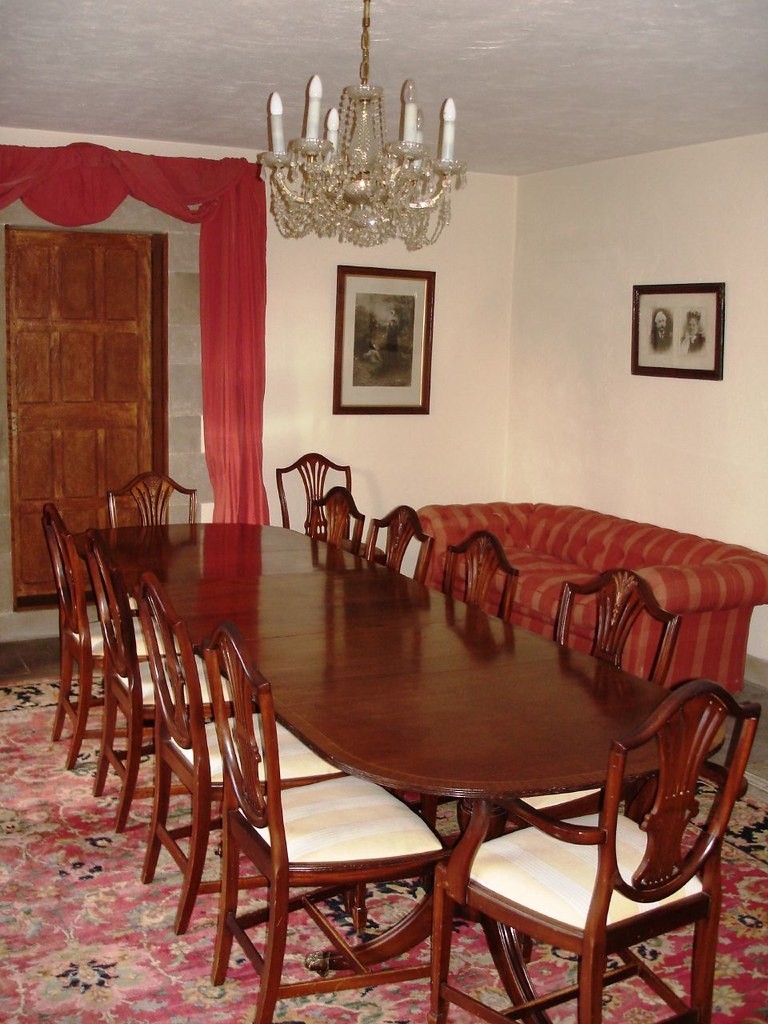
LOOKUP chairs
[41,454,762,1022]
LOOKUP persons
[650,310,672,354]
[680,310,706,355]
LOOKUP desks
[71,523,727,1023]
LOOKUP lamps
[258,0,468,251]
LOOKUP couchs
[416,502,768,691]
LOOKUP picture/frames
[631,283,726,380]
[333,265,436,415]
